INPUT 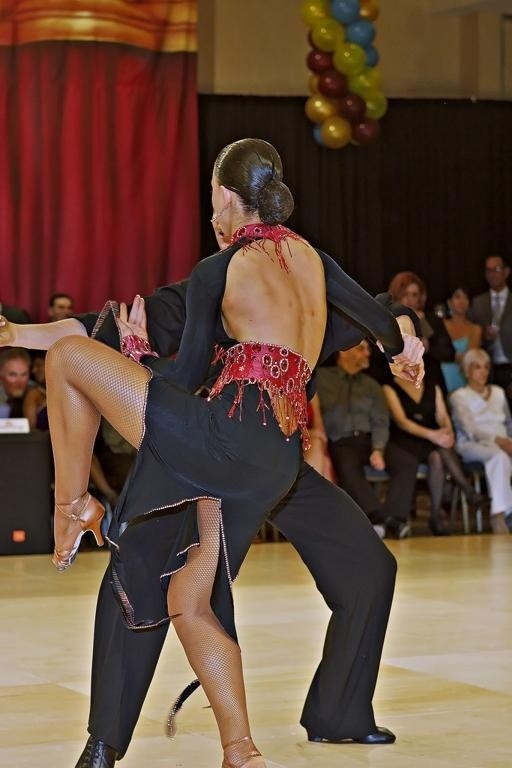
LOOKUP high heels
[51,491,106,571]
[222,736,268,768]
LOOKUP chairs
[357,363,490,531]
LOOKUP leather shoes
[75,734,119,768]
[467,497,492,506]
[427,516,450,536]
[307,726,396,744]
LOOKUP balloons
[305,2,390,150]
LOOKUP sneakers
[389,518,410,538]
[372,523,388,540]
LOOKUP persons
[1,211,420,767]
[2,253,510,540]
[44,138,427,767]
